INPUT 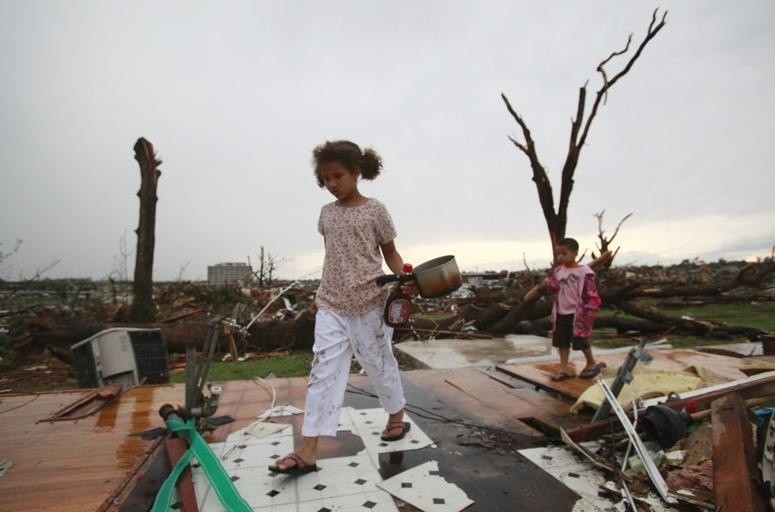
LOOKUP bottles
[382,263,413,329]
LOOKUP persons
[545,238,606,381]
[269,139,415,475]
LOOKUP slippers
[551,372,576,381]
[580,362,607,378]
[269,453,316,475]
[382,421,410,441]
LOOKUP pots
[375,254,463,300]
